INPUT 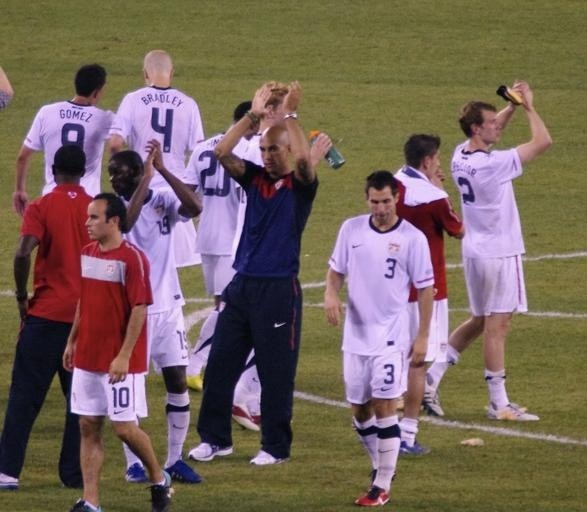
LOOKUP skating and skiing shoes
[124,461,149,483]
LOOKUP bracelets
[246,107,258,124]
[279,111,297,120]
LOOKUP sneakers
[248,449,291,466]
[149,469,172,511]
[230,402,262,431]
[68,496,102,512]
[186,440,234,463]
[185,372,205,393]
[0,471,20,490]
[353,466,396,507]
[481,400,541,422]
[398,437,431,457]
[423,375,445,417]
[161,458,203,484]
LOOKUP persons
[62,192,175,511]
[181,99,258,391]
[105,51,204,269]
[107,141,206,484]
[12,61,113,215]
[323,170,436,506]
[187,80,318,465]
[394,134,465,457]
[0,143,95,487]
[423,76,553,421]
[228,81,332,432]
[0,66,14,110]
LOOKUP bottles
[307,130,346,170]
